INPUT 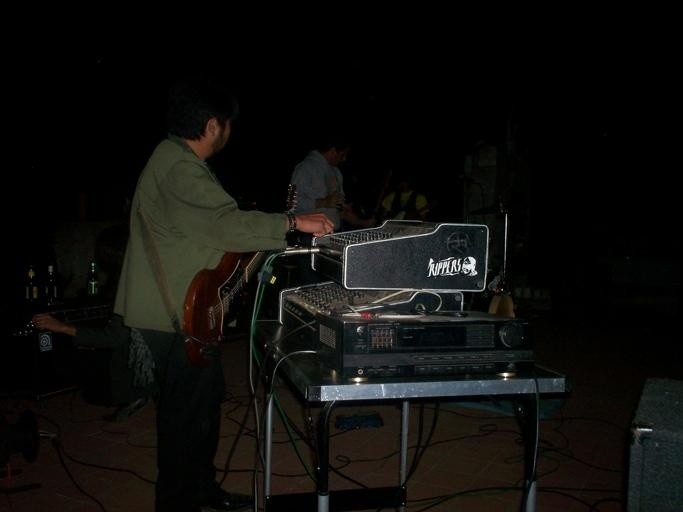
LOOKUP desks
[255,319,567,510]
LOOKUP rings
[36,321,39,325]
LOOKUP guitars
[11,303,115,340]
[183,183,298,372]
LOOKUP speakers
[625,378,682,512]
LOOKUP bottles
[38,329,54,354]
[43,265,59,307]
[86,256,100,300]
[23,264,40,307]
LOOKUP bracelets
[284,212,292,232]
[290,213,296,233]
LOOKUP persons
[31,311,148,423]
[339,187,388,230]
[110,91,334,510]
[284,132,352,287]
[377,168,433,230]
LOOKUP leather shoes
[109,397,146,419]
[206,486,252,512]
